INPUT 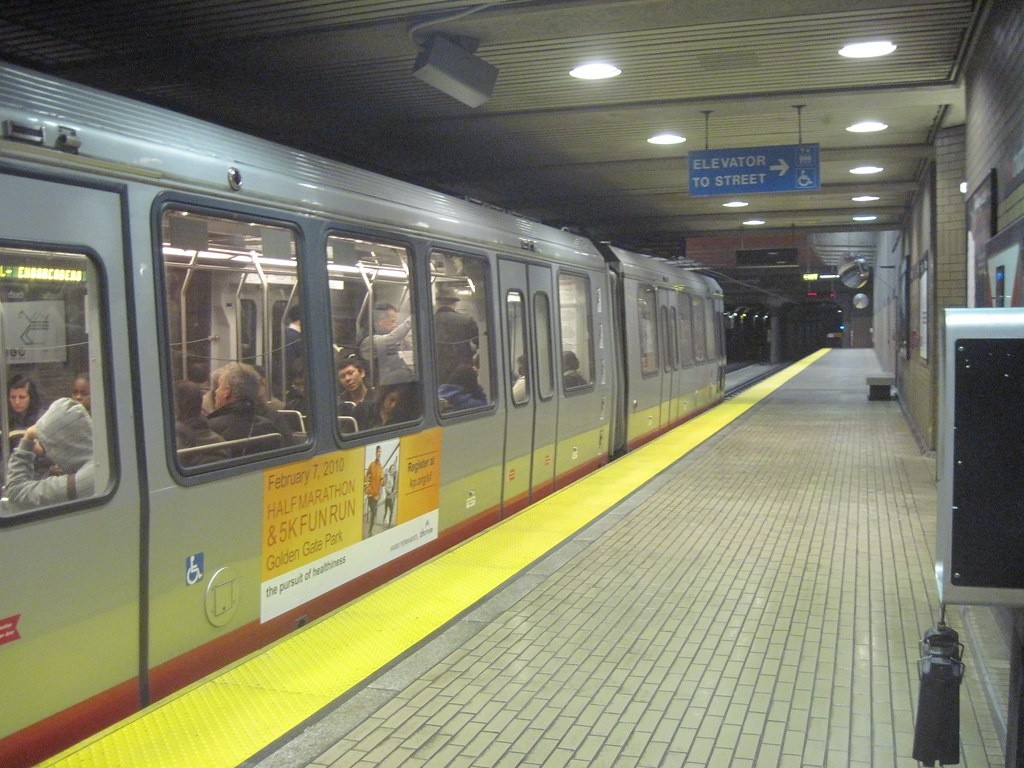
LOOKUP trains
[0,61,728,767]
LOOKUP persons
[383,464,398,529]
[364,446,384,536]
[5,285,587,510]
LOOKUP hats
[562,350,580,369]
[435,287,462,302]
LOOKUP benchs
[270,400,360,444]
[865,369,897,400]
